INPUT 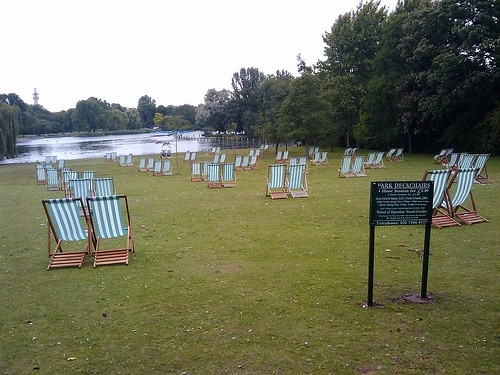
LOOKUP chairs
[35,145,493,270]
